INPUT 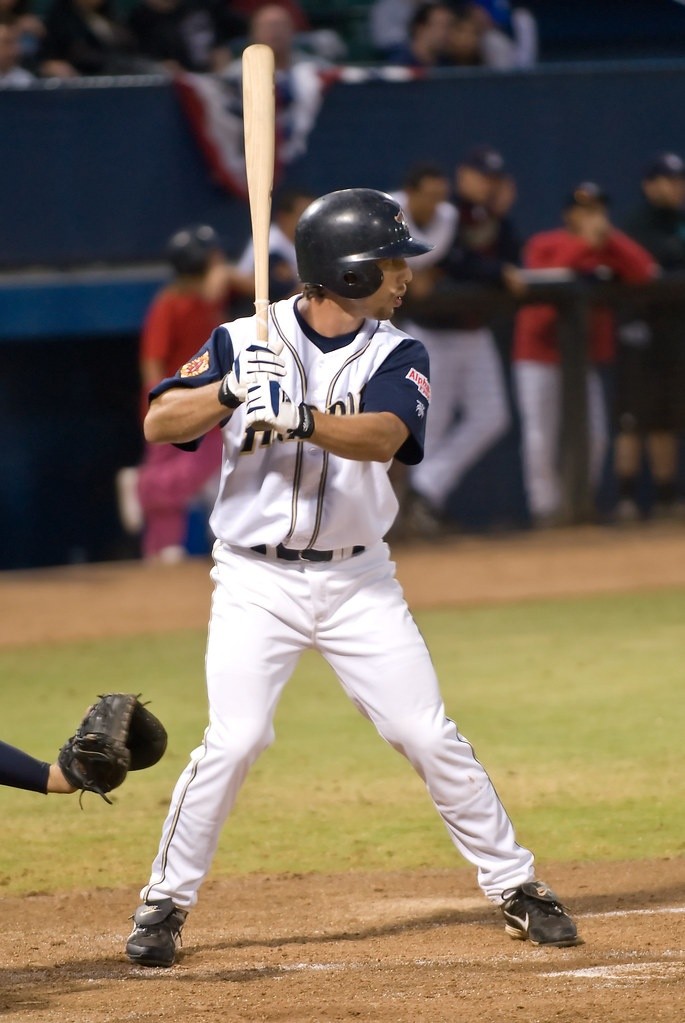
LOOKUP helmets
[296,187,433,299]
[169,227,229,277]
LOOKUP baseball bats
[238,41,279,435]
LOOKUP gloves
[219,340,286,408]
[244,381,314,440]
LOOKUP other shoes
[118,467,142,530]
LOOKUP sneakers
[501,881,579,947]
[124,898,188,965]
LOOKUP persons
[387,148,685,540]
[0,0,685,88]
[125,187,579,966]
[0,692,167,810]
[116,189,315,565]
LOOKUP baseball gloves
[57,689,170,814]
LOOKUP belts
[250,544,364,561]
